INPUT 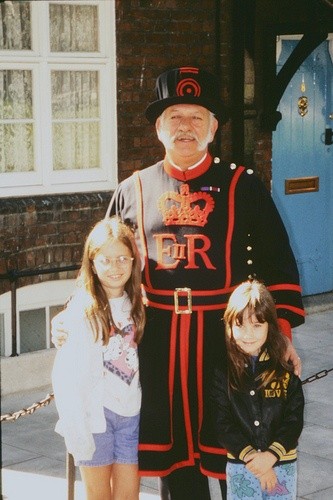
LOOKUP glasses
[89,256,135,267]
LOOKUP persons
[212,280,305,500]
[52,218,145,500]
[50,67,307,500]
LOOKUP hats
[143,66,233,129]
